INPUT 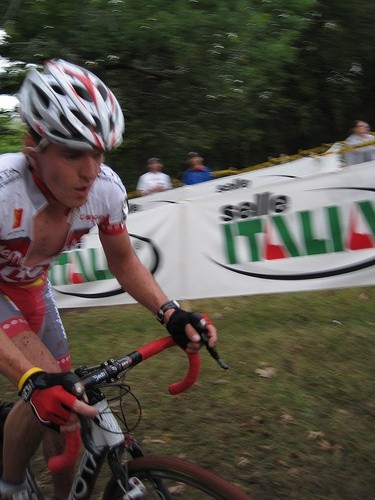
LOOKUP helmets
[16,56,125,152]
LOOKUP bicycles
[0,307,254,500]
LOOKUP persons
[347,120,374,147]
[182,152,211,185]
[0,59,218,499]
[135,158,172,195]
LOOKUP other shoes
[0,478,37,500]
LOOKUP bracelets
[156,300,180,325]
